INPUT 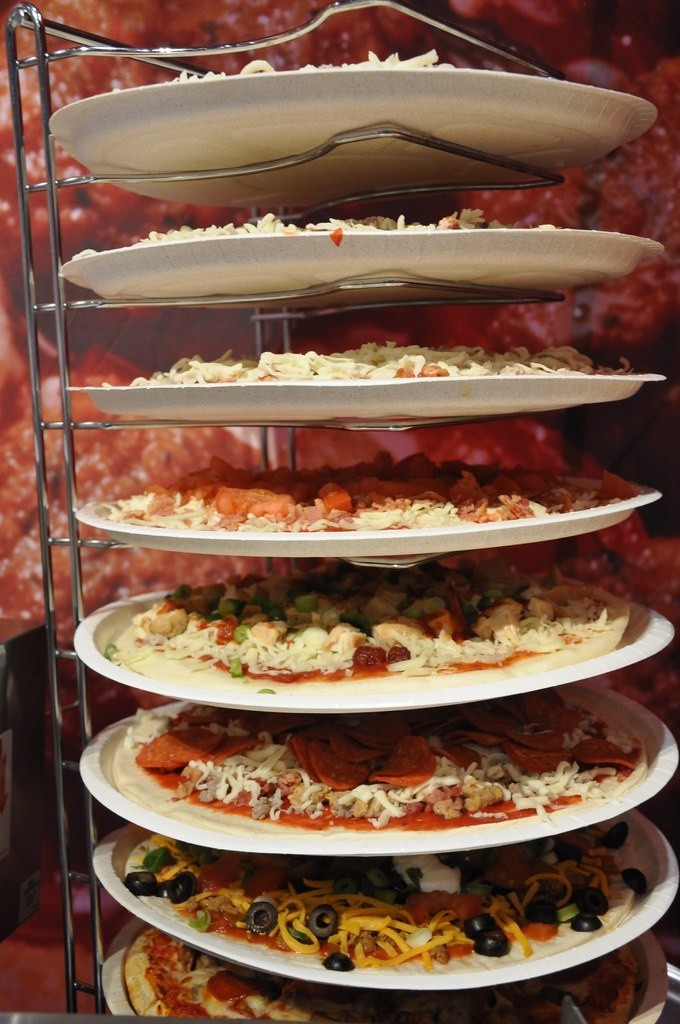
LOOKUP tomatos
[140,449,641,514]
[134,708,637,791]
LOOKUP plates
[74,476,662,558]
[48,65,658,208]
[58,229,665,309]
[66,373,667,421]
[77,682,679,857]
[72,579,675,714]
[91,825,679,990]
[101,917,668,1024]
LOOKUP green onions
[142,841,581,945]
[95,565,532,694]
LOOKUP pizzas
[124,922,639,1024]
